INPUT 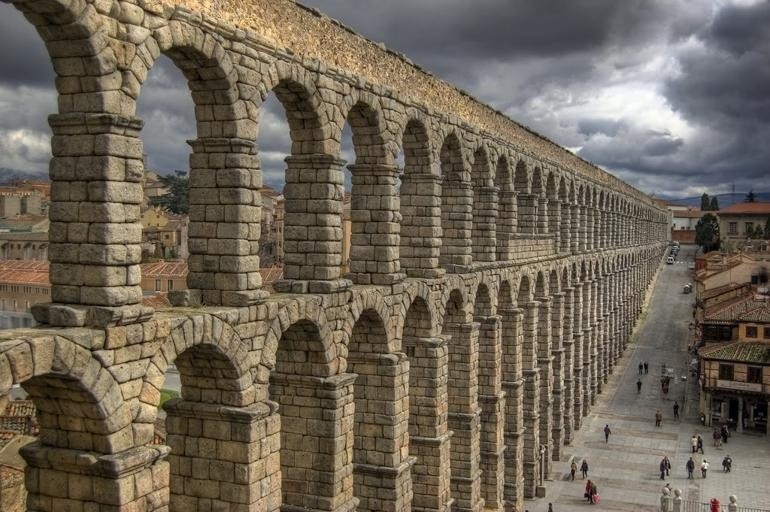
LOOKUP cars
[666,241,681,264]
[689,358,700,374]
[694,340,705,355]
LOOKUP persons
[526,359,732,512]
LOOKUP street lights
[537,444,548,498]
